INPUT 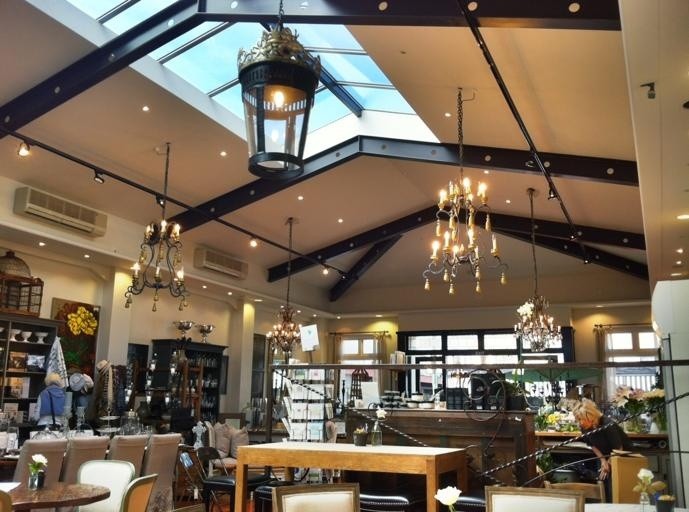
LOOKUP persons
[33,372,73,432]
[556,385,581,412]
[572,397,634,504]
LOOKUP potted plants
[492,358,535,411]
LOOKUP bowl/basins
[406,393,436,409]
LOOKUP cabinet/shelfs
[152,339,229,432]
[0,312,65,444]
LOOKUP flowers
[609,385,646,431]
[643,388,667,431]
[371,408,388,442]
[53,301,98,372]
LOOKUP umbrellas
[44,335,69,390]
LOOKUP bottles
[188,357,219,422]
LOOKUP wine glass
[0,326,49,344]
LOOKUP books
[271,367,347,488]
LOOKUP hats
[96,360,112,375]
[69,372,94,395]
